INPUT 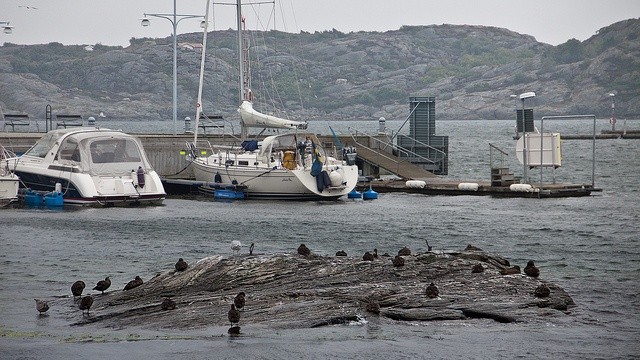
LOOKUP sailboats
[186,1,359,201]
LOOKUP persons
[303,136,313,145]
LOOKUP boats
[0,127,167,207]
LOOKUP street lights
[520,91,536,184]
[141,14,206,135]
[609,93,616,132]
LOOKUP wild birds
[174,258,188,272]
[122,276,143,291]
[71,280,85,298]
[524,261,539,277]
[92,277,111,293]
[336,250,347,256]
[393,256,404,266]
[34,298,49,314]
[535,284,550,298]
[426,282,439,298]
[79,295,93,314]
[161,298,176,311]
[363,249,378,261]
[234,292,245,310]
[249,242,255,255]
[471,263,484,273]
[398,246,411,256]
[231,240,242,256]
[228,304,240,326]
[298,244,310,256]
